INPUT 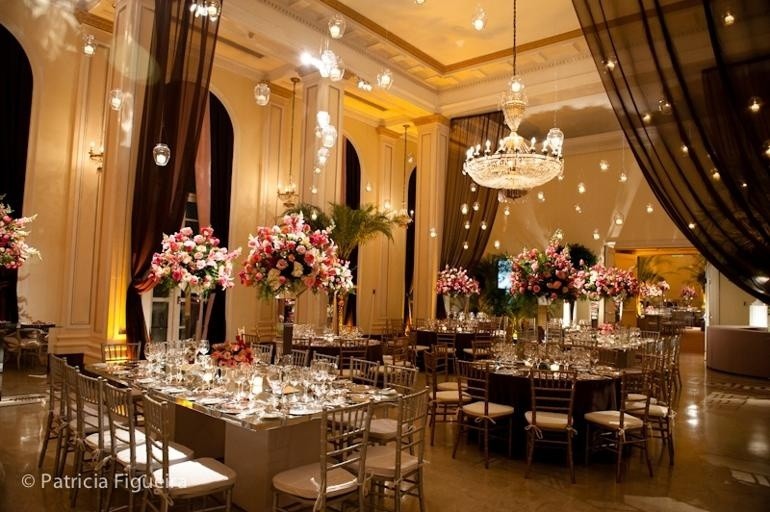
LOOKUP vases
[449,295,455,316]
[185,293,200,346]
[545,301,561,354]
[464,295,470,316]
[590,302,599,331]
[613,298,623,324]
[282,301,293,362]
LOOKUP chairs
[101,342,142,361]
[624,356,676,478]
[326,356,381,461]
[291,337,313,351]
[338,336,370,366]
[255,320,274,342]
[241,333,260,344]
[312,351,340,366]
[583,369,656,482]
[624,349,669,405]
[3,328,45,368]
[250,342,275,363]
[341,385,431,511]
[56,361,129,485]
[69,370,158,512]
[477,321,492,340]
[39,352,109,482]
[416,318,427,328]
[522,368,579,485]
[101,379,195,512]
[436,325,456,348]
[423,341,448,387]
[423,355,471,447]
[291,349,310,366]
[639,332,660,353]
[379,317,417,364]
[140,389,238,512]
[451,360,514,468]
[273,395,375,512]
[348,362,419,457]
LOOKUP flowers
[0,195,41,270]
[433,264,480,299]
[638,270,671,300]
[580,264,610,302]
[680,285,698,300]
[212,335,253,366]
[509,239,591,304]
[602,264,639,300]
[239,208,355,300]
[151,226,242,299]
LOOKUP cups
[495,343,600,371]
[561,326,640,342]
[132,340,353,417]
[293,323,362,343]
[423,313,493,333]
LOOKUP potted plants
[278,198,408,331]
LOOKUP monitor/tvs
[494,257,511,290]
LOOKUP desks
[276,336,382,369]
[459,361,644,471]
[84,360,400,512]
[412,326,491,373]
[532,322,655,366]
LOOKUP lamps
[82,29,95,57]
[356,79,373,93]
[472,8,486,30]
[328,13,348,40]
[188,2,223,24]
[277,183,298,208]
[312,111,337,175]
[298,36,346,82]
[748,299,768,330]
[461,1,565,191]
[376,13,393,90]
[108,89,122,111]
[88,141,104,171]
[152,1,171,167]
[253,81,272,107]
